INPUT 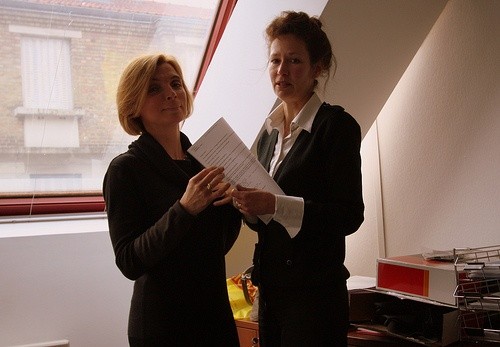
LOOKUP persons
[102,54,244,347]
[212,8,365,347]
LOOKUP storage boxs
[375,254,500,306]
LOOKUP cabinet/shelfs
[346,327,500,347]
[235,318,260,347]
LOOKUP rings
[235,200,242,210]
[206,183,215,191]
